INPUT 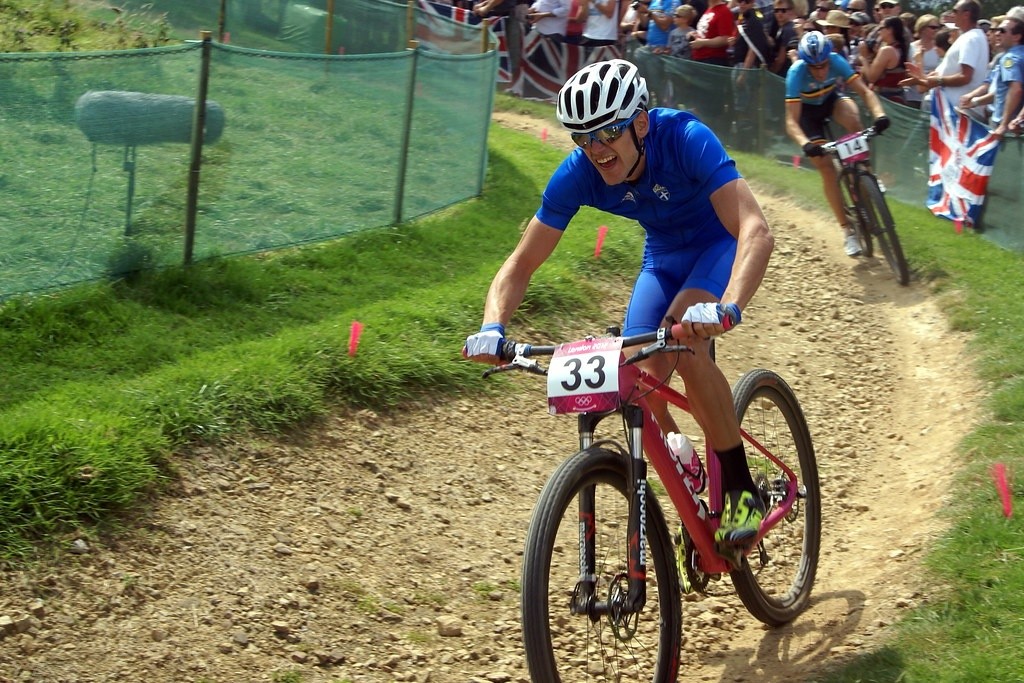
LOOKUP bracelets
[938,77,944,85]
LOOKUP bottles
[847,173,859,203]
[667,431,709,493]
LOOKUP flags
[926,87,1001,228]
[417,1,622,98]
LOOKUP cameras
[850,37,877,51]
[632,2,640,10]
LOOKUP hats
[849,12,871,24]
[816,10,852,29]
[879,0,901,6]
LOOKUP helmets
[556,59,648,132]
[798,31,833,62]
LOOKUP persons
[466,60,773,595]
[784,32,890,256]
[433,0,1024,192]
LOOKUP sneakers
[715,486,765,543]
[843,234,862,258]
[674,522,701,594]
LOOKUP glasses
[995,28,1007,34]
[879,26,887,30]
[773,8,790,14]
[672,13,684,19]
[737,0,752,4]
[873,9,880,12]
[809,60,830,69]
[880,4,894,9]
[952,9,960,13]
[928,26,941,30]
[570,110,644,149]
[847,7,865,14]
[816,6,827,12]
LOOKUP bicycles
[460,303,826,683]
[790,115,912,286]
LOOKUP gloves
[874,115,891,132]
[802,142,821,157]
